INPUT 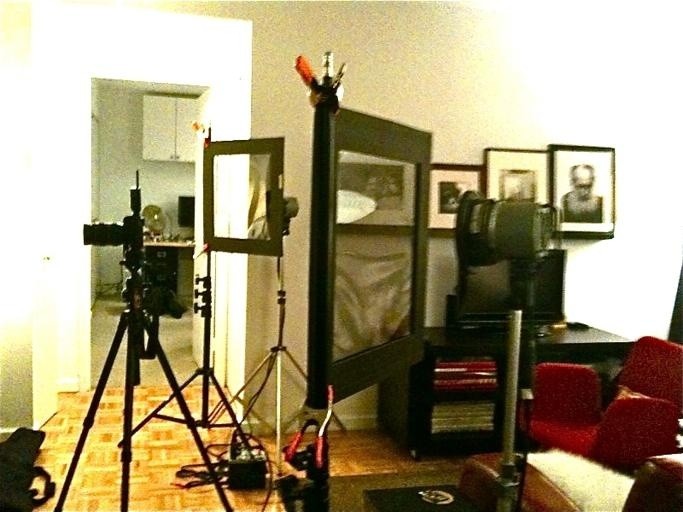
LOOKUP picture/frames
[331,144,615,239]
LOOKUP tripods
[53,305,233,512]
[118,251,252,450]
[211,259,345,477]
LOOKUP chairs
[519,336,683,471]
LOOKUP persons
[561,164,603,222]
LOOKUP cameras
[455,188,557,265]
[83,169,144,258]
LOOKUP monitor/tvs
[453,250,566,325]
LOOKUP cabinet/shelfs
[141,247,178,295]
[141,92,197,164]
[375,315,635,463]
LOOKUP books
[431,357,499,433]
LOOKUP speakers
[178,196,193,227]
